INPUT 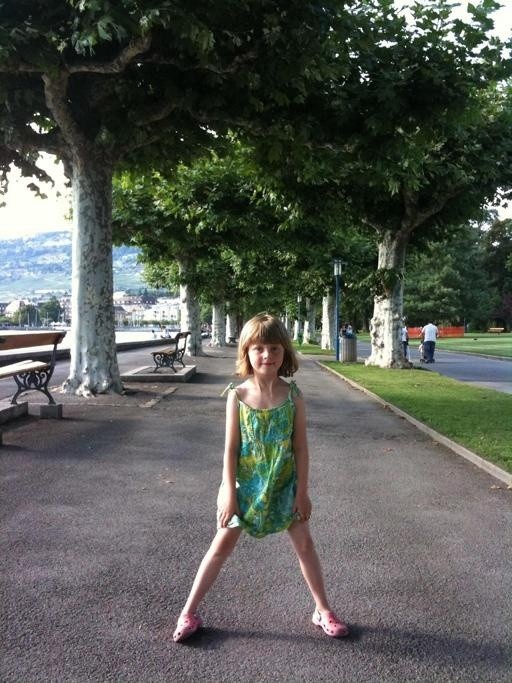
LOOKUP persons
[418,340,423,355]
[169,310,353,643]
[401,320,408,358]
[346,324,352,335]
[340,324,346,336]
[421,318,439,361]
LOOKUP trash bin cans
[339,333,358,363]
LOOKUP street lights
[297,293,302,344]
[333,253,342,362]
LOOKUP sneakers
[312,606,349,638]
[173,611,203,642]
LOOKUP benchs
[0,329,66,424]
[150,329,193,373]
